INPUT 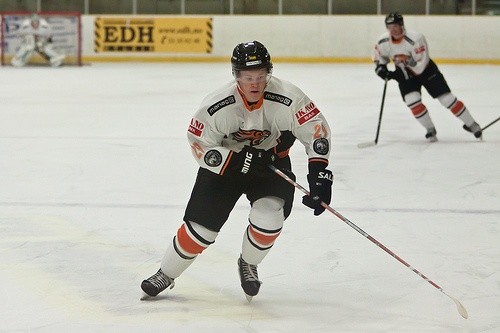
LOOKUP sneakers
[140,268,175,300]
[471,122,482,141]
[425,127,437,142]
[237,253,263,303]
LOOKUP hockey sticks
[464,116,500,133]
[357,77,389,147]
[267,163,469,320]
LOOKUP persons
[140,41,332,302]
[11,14,64,67]
[375,12,482,143]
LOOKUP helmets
[385,11,403,29]
[231,41,273,76]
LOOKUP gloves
[375,64,393,81]
[302,170,333,216]
[392,65,416,81]
[231,144,267,177]
